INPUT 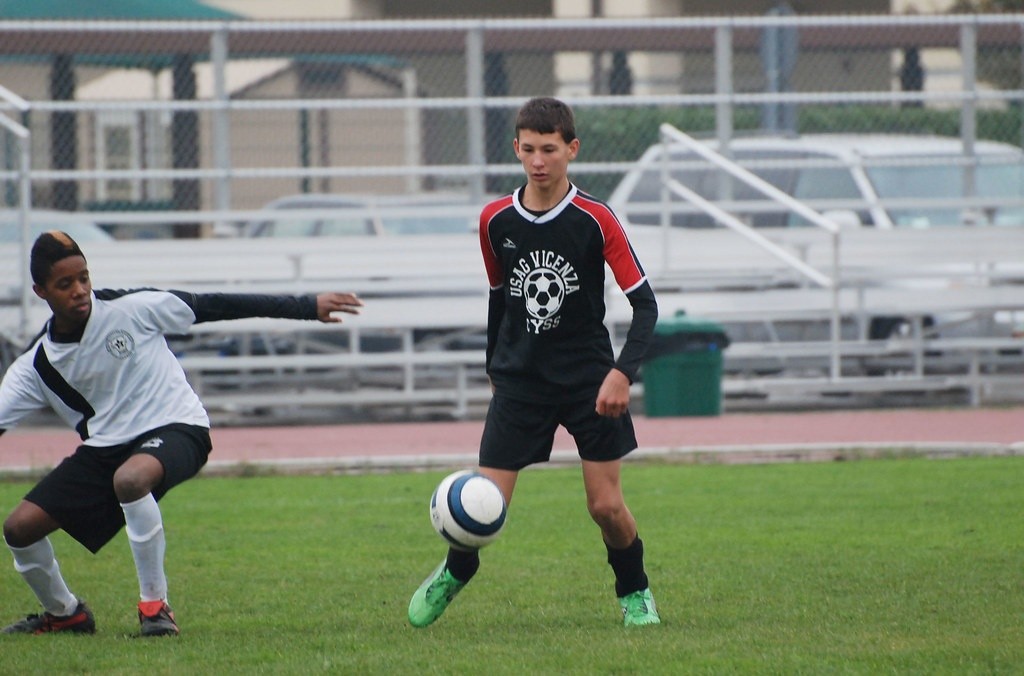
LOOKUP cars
[597,132,1024,400]
[208,187,501,364]
[0,204,116,386]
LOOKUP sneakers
[2,598,96,636]
[617,585,661,629]
[407,555,480,629]
[136,597,179,636]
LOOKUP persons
[0,231,365,634]
[407,99,660,630]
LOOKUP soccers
[428,470,506,548]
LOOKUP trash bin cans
[642,319,732,418]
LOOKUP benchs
[0,219,1024,424]
